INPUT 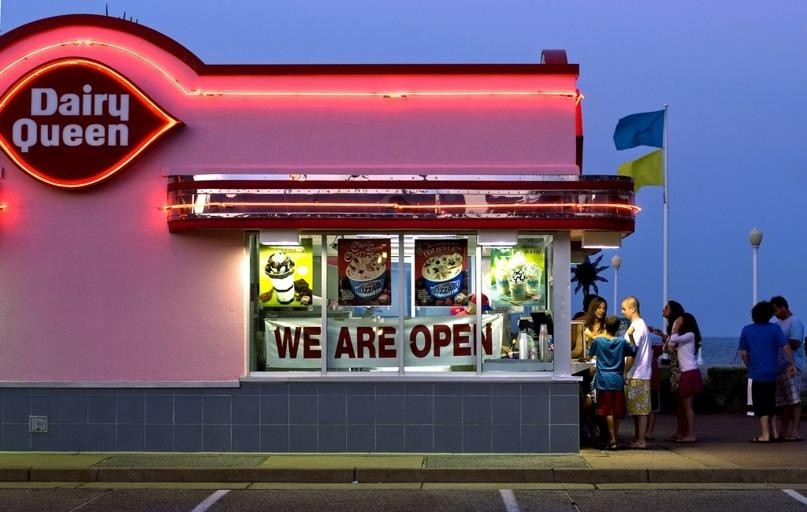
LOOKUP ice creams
[266,250,293,274]
[509,269,527,285]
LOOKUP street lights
[611,253,622,316]
[749,227,765,307]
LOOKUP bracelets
[629,335,634,339]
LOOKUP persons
[771,295,806,441]
[570,297,607,439]
[570,294,598,350]
[654,300,685,442]
[586,315,638,450]
[739,301,797,444]
[621,296,654,450]
[668,313,704,443]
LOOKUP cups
[345,263,386,299]
[539,324,550,361]
[421,258,465,299]
[265,260,297,304]
[495,266,542,301]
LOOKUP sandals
[602,430,697,450]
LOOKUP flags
[617,149,664,192]
[612,109,664,150]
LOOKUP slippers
[748,432,804,444]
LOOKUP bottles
[519,330,530,359]
